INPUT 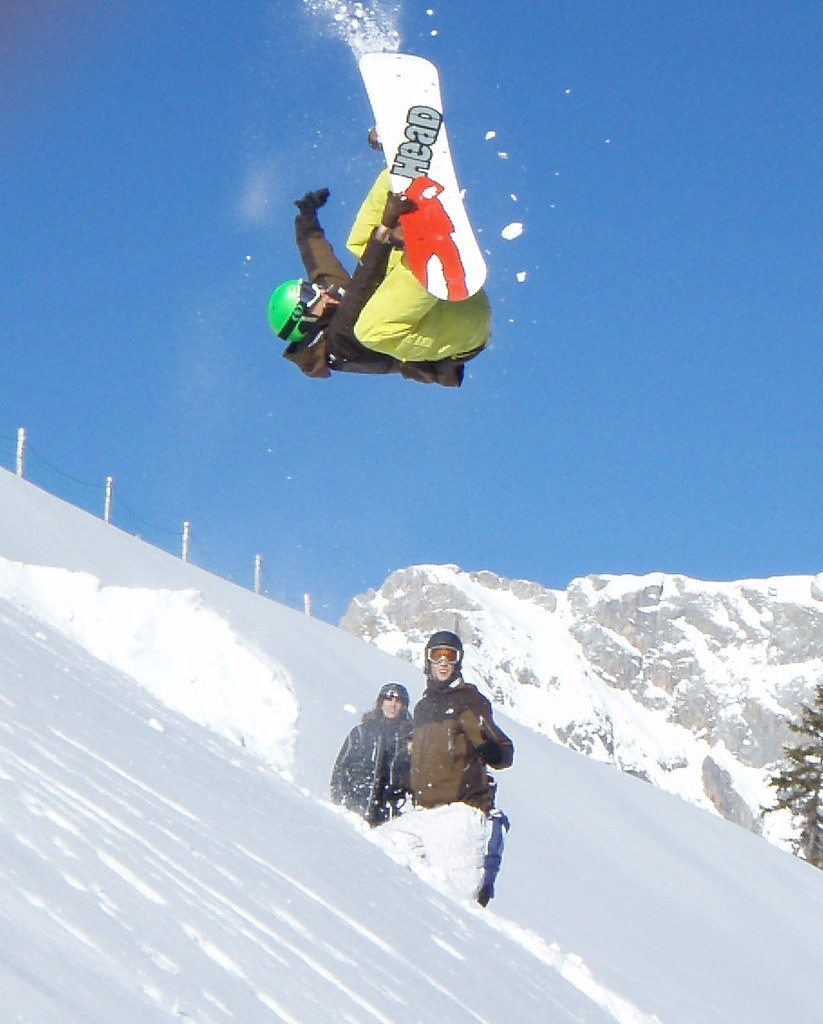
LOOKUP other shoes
[368,128,383,152]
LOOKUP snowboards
[356,50,489,304]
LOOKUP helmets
[424,631,464,675]
[485,766,497,793]
[379,683,409,704]
[267,280,320,344]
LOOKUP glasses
[486,774,496,786]
[427,645,460,665]
[278,280,320,341]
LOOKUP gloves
[294,187,330,215]
[476,731,503,768]
[381,191,418,229]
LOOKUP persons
[330,632,514,908]
[267,128,492,388]
